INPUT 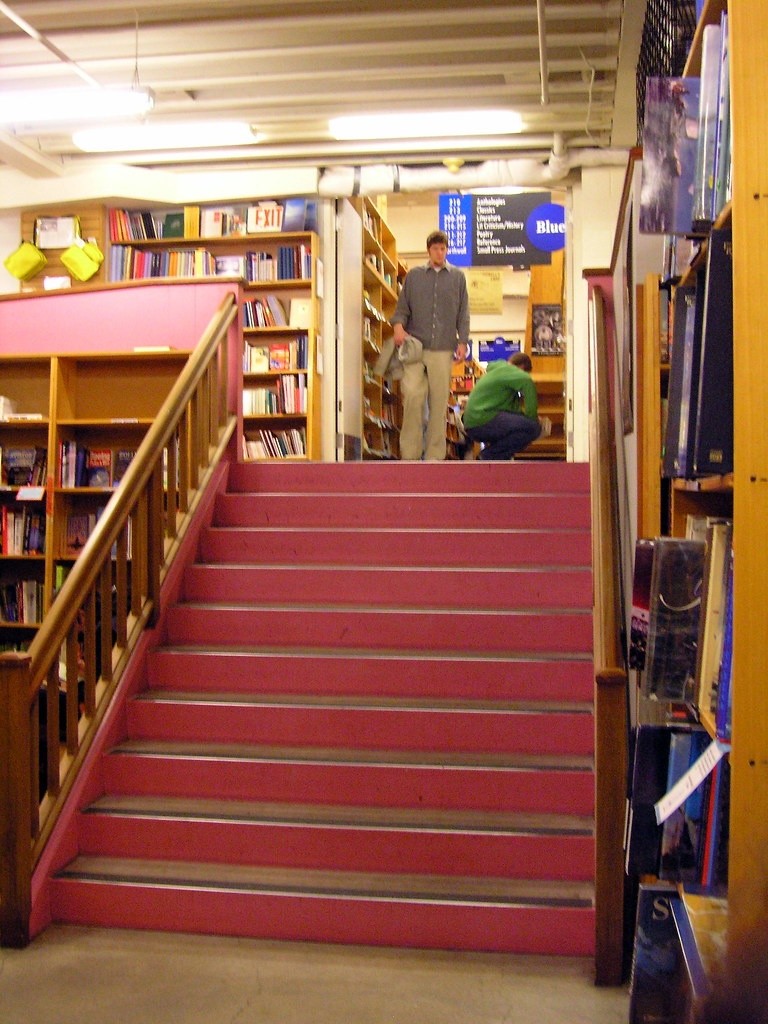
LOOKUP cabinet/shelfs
[0,195,483,688]
[582,0,768,1024]
[513,248,572,457]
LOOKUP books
[622,0,734,1024]
[0,199,481,812]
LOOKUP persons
[463,354,542,461]
[390,231,469,459]
